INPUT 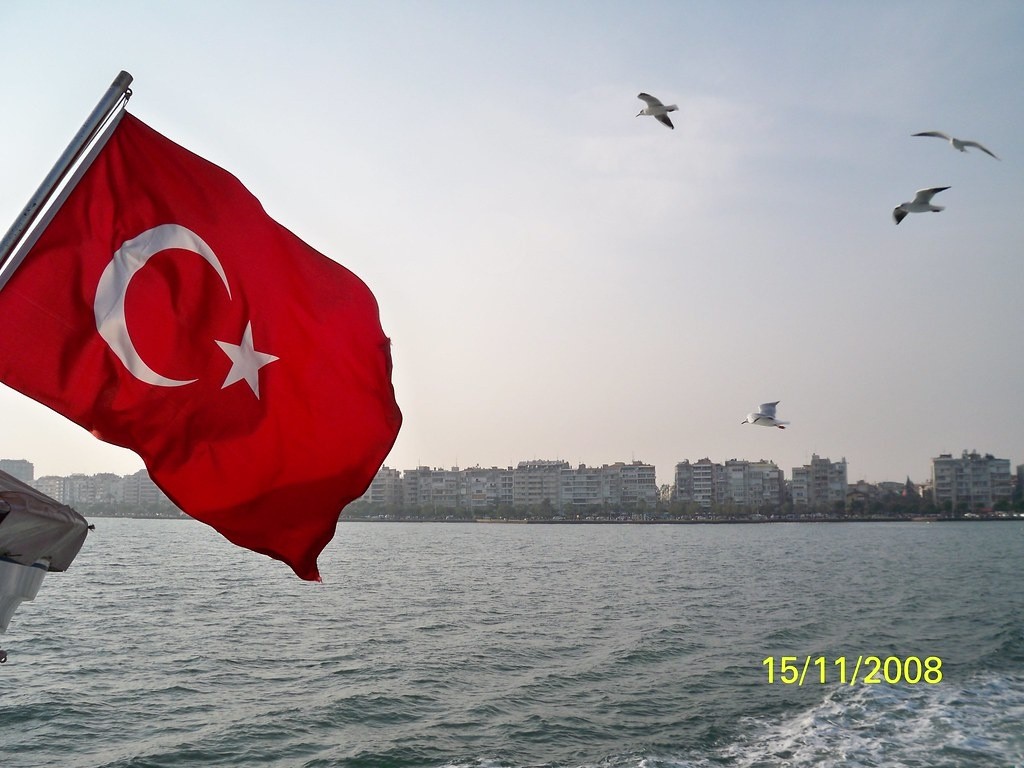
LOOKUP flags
[0,106,404,582]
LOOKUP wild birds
[891,188,949,226]
[638,93,679,130]
[913,131,999,161]
[741,403,789,433]
